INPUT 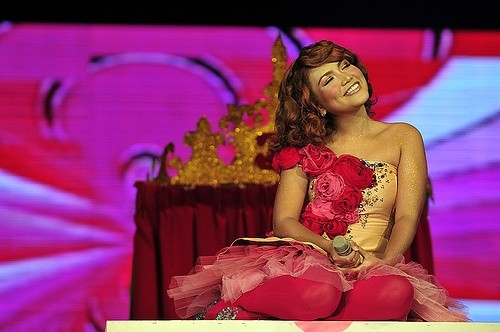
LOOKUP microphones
[332,235,353,256]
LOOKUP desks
[130,179,439,320]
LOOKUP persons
[167,40,472,321]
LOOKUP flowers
[270,143,373,237]
